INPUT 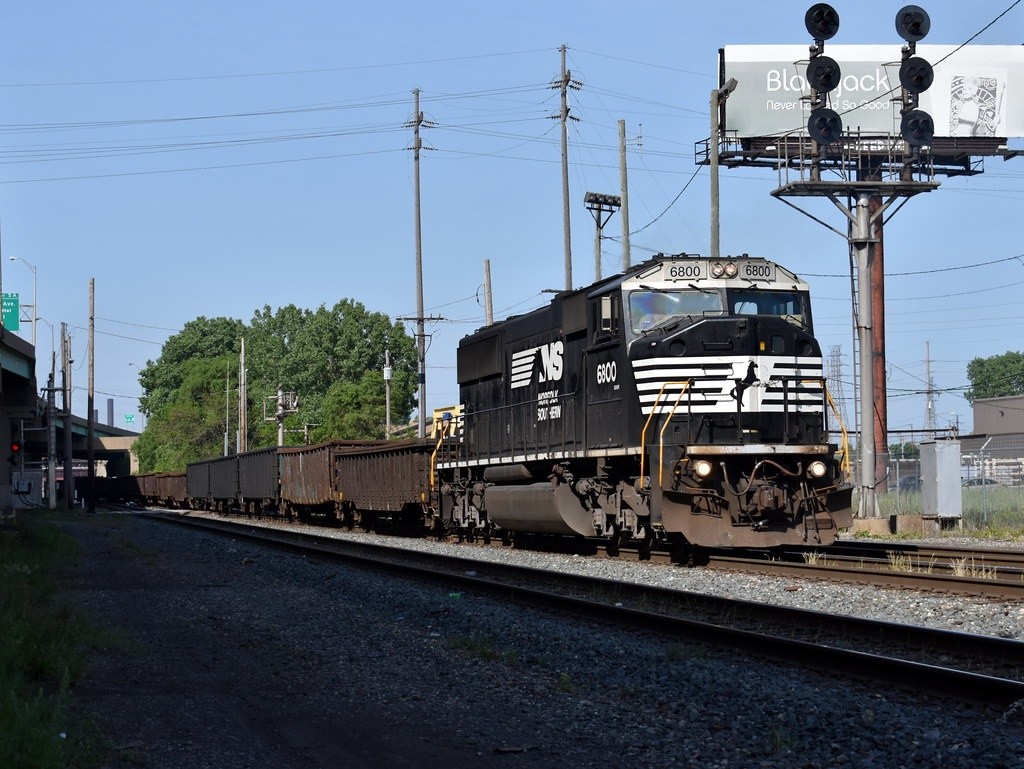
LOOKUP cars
[962,478,1008,493]
[887,474,920,492]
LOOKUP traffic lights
[808,109,842,144]
[11,441,20,453]
[901,109,934,146]
[895,4,931,42]
[804,3,839,41]
[807,56,841,93]
[899,58,934,94]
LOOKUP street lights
[584,191,621,283]
[11,255,37,346]
[710,78,739,256]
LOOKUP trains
[74,249,856,566]
